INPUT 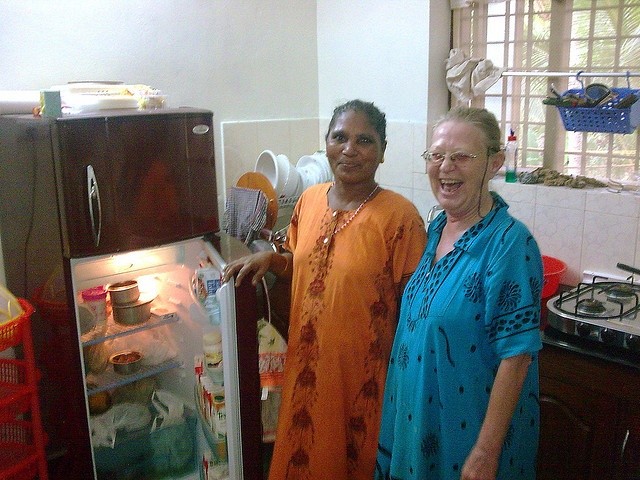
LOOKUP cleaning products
[505,128,518,183]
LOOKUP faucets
[426,205,443,224]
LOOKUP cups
[583,83,612,106]
[81,285,108,326]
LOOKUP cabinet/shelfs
[0,298,49,480]
[537,347,640,480]
[275,195,299,209]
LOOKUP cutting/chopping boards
[231,170,278,230]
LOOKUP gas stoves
[546,276,640,356]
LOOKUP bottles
[202,333,223,374]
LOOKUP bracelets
[276,259,289,278]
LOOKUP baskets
[0,427,36,479]
[3,361,41,422]
[0,302,35,352]
[557,87,640,134]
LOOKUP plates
[293,149,335,198]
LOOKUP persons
[221,99,429,479]
[373,105,594,479]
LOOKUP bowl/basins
[109,351,144,374]
[77,303,98,336]
[276,154,298,200]
[107,279,141,306]
[112,302,151,326]
[254,150,287,199]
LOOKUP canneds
[203,331,223,371]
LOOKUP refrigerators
[0,103,265,480]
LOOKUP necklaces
[329,181,380,235]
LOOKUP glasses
[420,147,492,166]
